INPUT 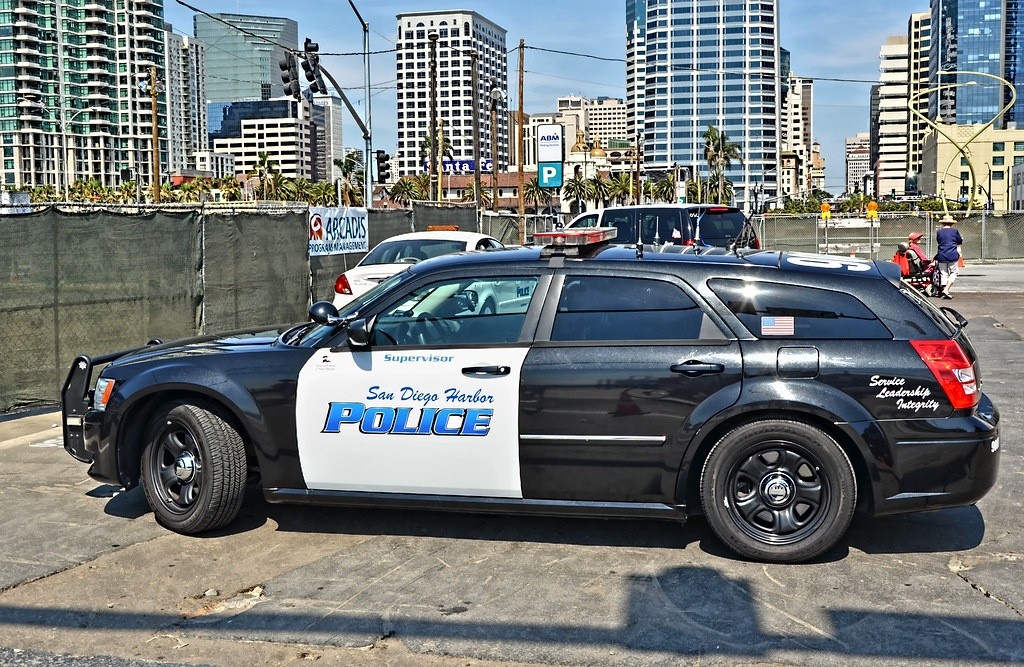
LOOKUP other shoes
[941,290,952,299]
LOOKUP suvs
[59,224,1002,565]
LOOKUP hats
[908,232,923,240]
[939,215,958,224]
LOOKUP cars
[330,224,540,347]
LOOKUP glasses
[916,237,921,240]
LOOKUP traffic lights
[892,189,895,200]
[301,53,328,94]
[278,50,300,98]
[376,149,391,184]
[305,37,319,52]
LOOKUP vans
[555,201,761,251]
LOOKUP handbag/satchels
[893,250,909,276]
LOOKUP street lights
[907,69,1017,218]
[427,31,441,200]
[16,95,103,202]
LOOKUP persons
[908,216,963,299]
